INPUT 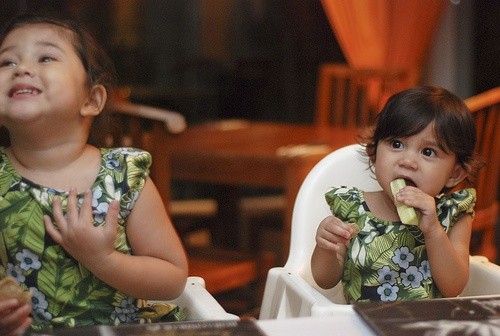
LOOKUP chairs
[259,143,500,320]
[259,145,329,310]
[89,101,276,317]
[463,87,500,263]
[315,65,385,130]
[149,277,240,322]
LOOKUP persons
[310,85,478,304]
[0,16,189,335]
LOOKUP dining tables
[26,294,499,336]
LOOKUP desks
[169,119,375,249]
